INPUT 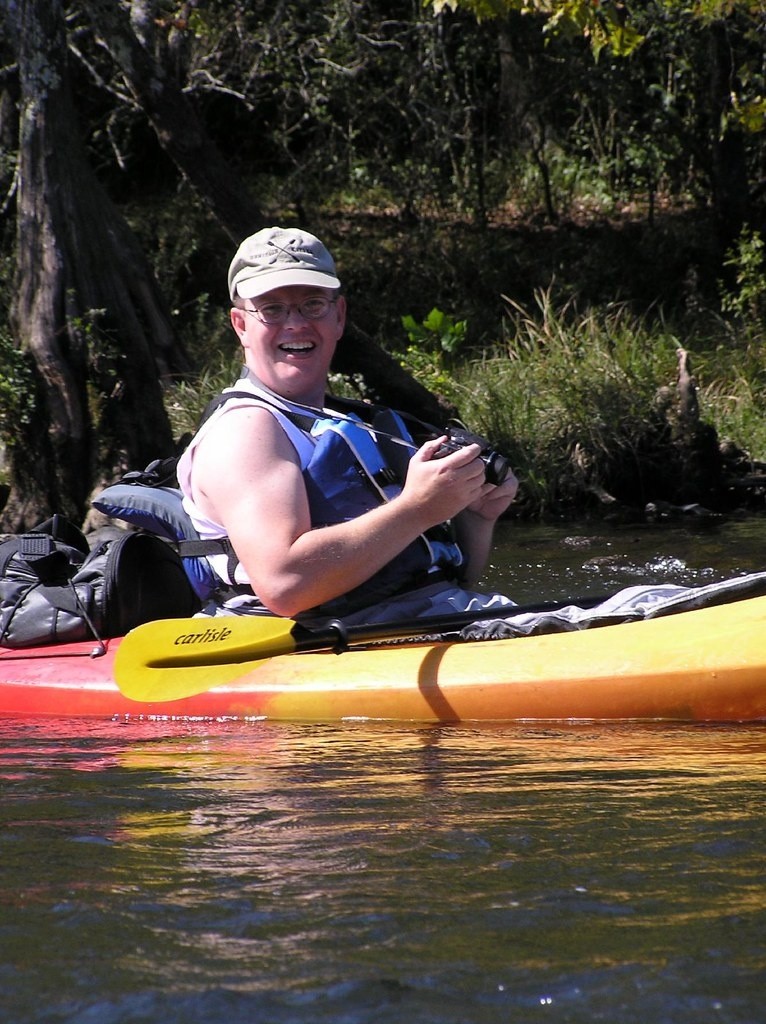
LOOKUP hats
[227,228,341,303]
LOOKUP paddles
[112,595,613,704]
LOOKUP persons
[177,227,518,640]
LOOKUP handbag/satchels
[0,513,202,650]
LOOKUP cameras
[433,427,509,487]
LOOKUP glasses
[241,296,337,324]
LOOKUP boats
[0,591,766,727]
